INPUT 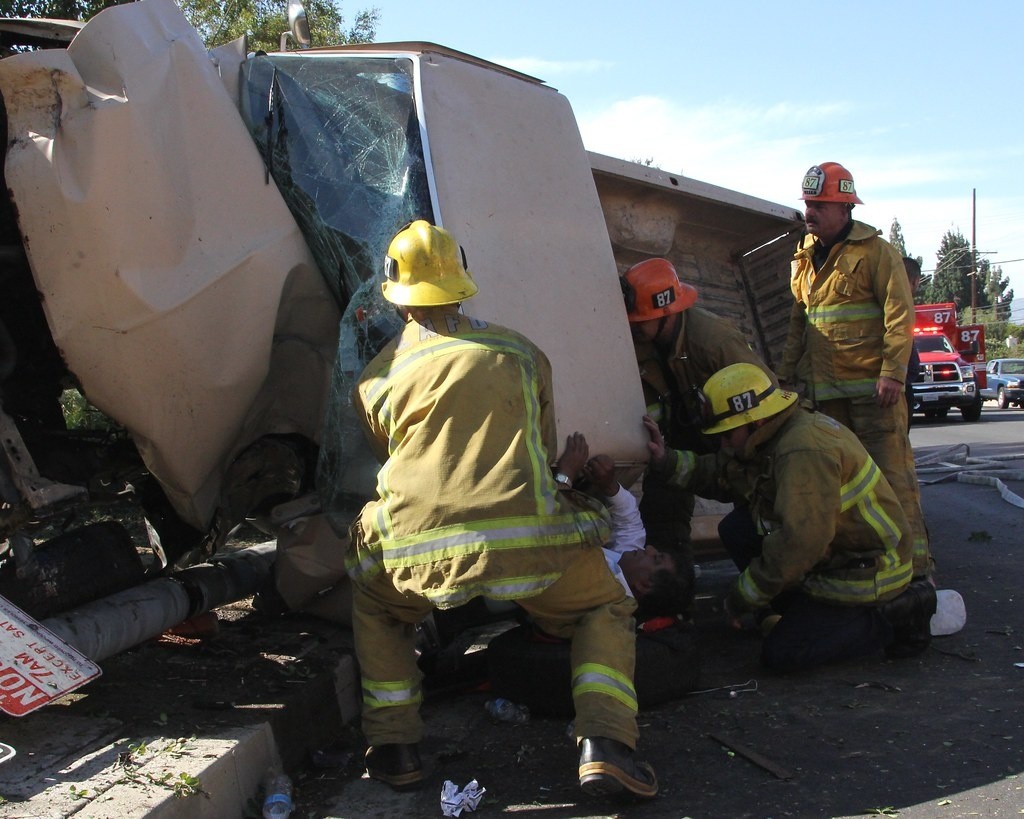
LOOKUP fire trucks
[908,302,989,424]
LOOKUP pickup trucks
[978,357,1024,409]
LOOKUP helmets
[624,258,698,323]
[697,363,799,434]
[799,161,863,204]
[381,218,480,306]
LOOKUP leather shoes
[885,580,937,661]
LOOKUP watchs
[556,473,572,488]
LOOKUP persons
[350,220,659,798]
[277,431,698,627]
[621,162,937,671]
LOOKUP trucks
[3,1,817,630]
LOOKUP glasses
[724,426,745,439]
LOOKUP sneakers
[365,741,421,789]
[579,735,658,801]
